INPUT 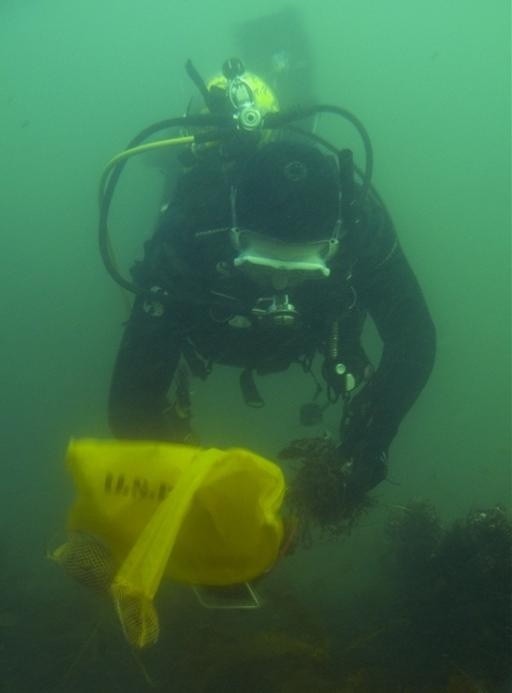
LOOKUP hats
[232,142,340,244]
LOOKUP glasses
[233,247,330,281]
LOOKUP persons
[107,12,438,528]
